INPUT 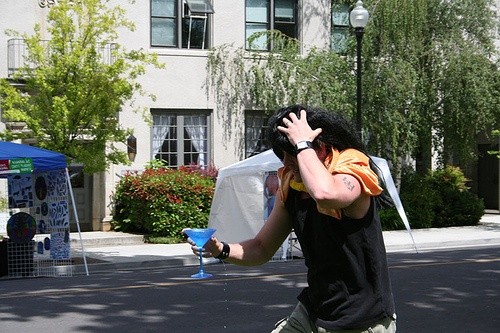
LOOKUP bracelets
[215,239,230,260]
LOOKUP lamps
[126,134,137,161]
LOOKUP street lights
[350,0,369,142]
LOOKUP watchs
[293,142,315,154]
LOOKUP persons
[180,105,398,333]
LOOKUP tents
[0,141,89,277]
[205,146,419,262]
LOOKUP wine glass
[184,229,216,278]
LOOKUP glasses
[273,143,296,159]
